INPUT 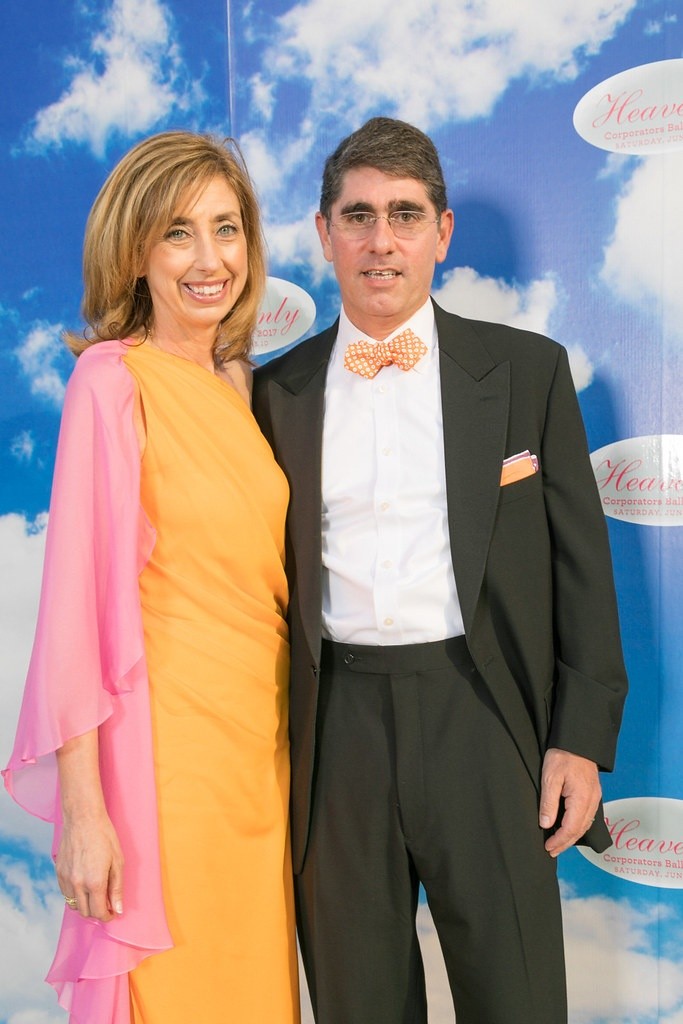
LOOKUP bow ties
[344,328,429,379]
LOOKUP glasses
[330,211,442,241]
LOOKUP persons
[1,128,298,1024]
[241,117,628,1024]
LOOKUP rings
[592,818,595,822]
[64,896,76,906]
